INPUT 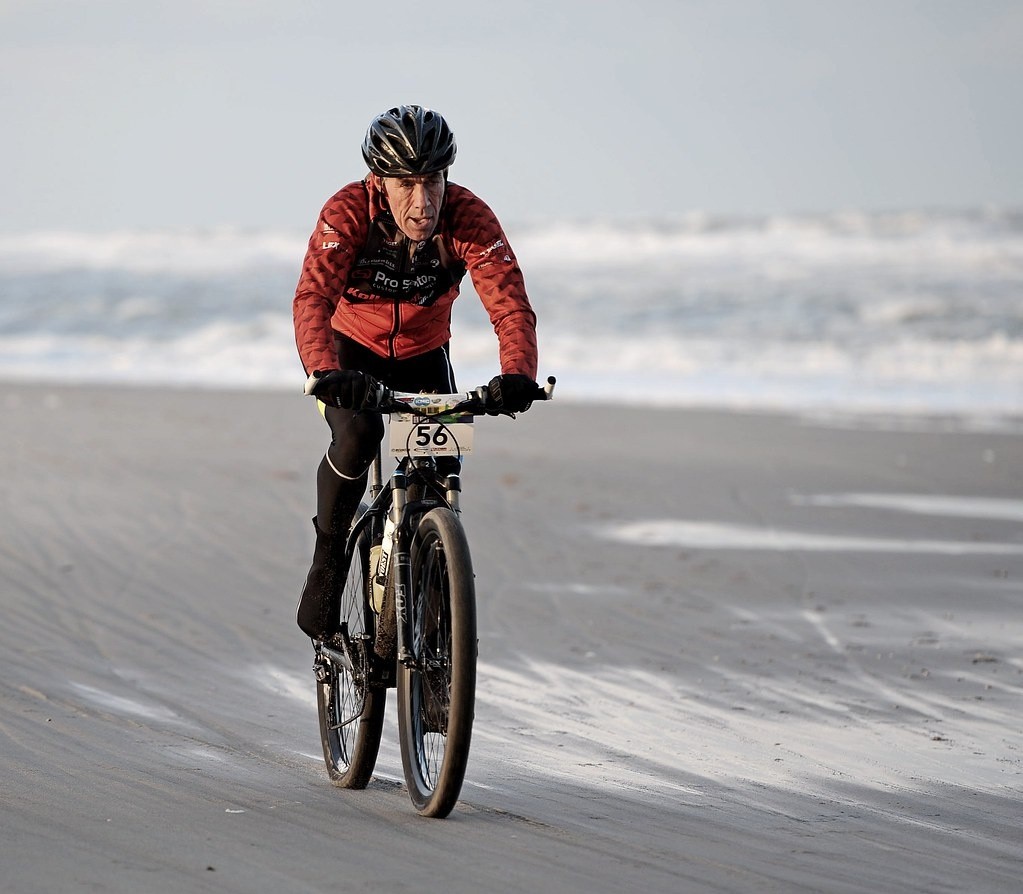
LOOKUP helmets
[361,104,457,178]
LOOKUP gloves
[315,370,378,413]
[488,373,538,420]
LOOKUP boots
[298,517,347,641]
[422,669,451,721]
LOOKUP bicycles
[300,364,556,819]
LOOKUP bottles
[377,509,394,575]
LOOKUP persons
[292,104,539,731]
